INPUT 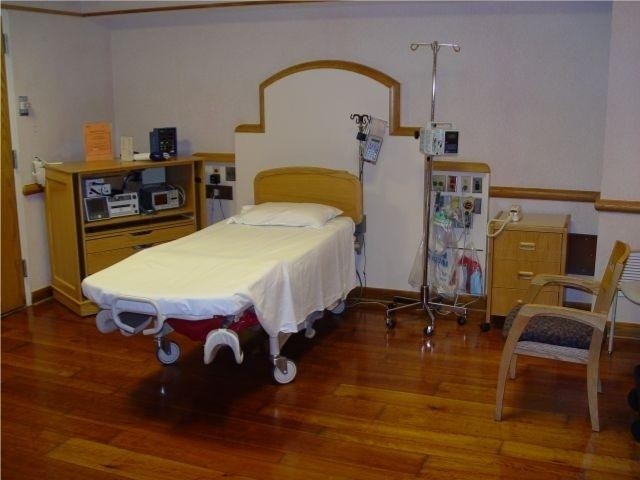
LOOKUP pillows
[227,202,346,229]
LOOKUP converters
[210,174,220,183]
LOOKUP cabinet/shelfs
[483,211,574,329]
[42,154,205,318]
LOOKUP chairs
[493,240,632,432]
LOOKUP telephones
[510,205,522,222]
[149,127,177,157]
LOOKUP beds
[74,168,364,384]
